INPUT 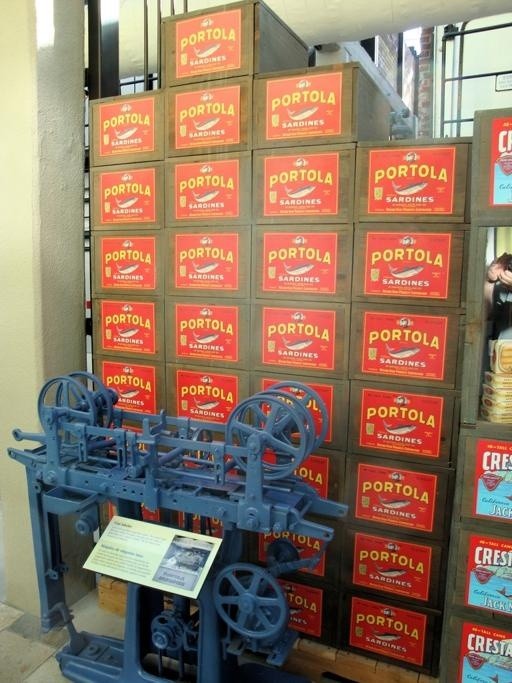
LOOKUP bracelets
[486,276,498,283]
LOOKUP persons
[483,251,511,339]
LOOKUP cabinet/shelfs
[90,96,167,620]
[159,1,311,85]
[446,421,511,683]
[252,60,476,673]
[473,107,512,234]
[168,90,244,541]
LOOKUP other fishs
[114,43,429,643]
